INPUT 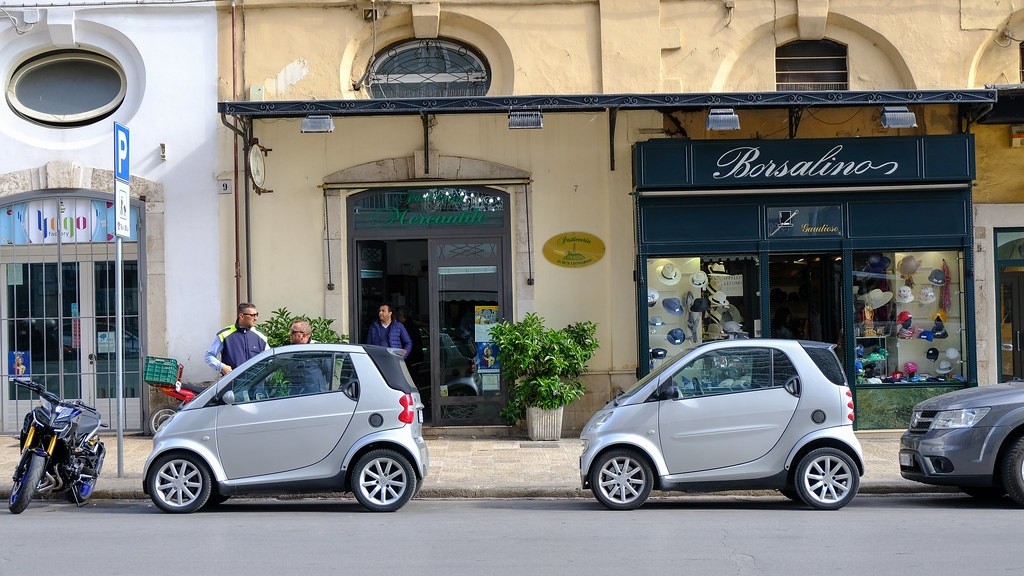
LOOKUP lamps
[880,107,917,127]
[508,108,544,129]
[301,111,334,132]
[709,109,740,129]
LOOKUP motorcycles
[8,379,110,514]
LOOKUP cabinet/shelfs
[852,251,897,381]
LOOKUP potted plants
[494,324,594,440]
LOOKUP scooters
[148,384,210,439]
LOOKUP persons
[366,304,412,358]
[397,306,423,380]
[282,320,336,395]
[204,302,275,396]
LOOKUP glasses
[290,331,305,336]
[243,313,259,317]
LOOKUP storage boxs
[143,355,178,386]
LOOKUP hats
[648,262,747,361]
[855,255,966,385]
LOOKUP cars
[436,329,484,420]
[141,344,429,516]
[577,340,866,513]
[898,380,1024,506]
